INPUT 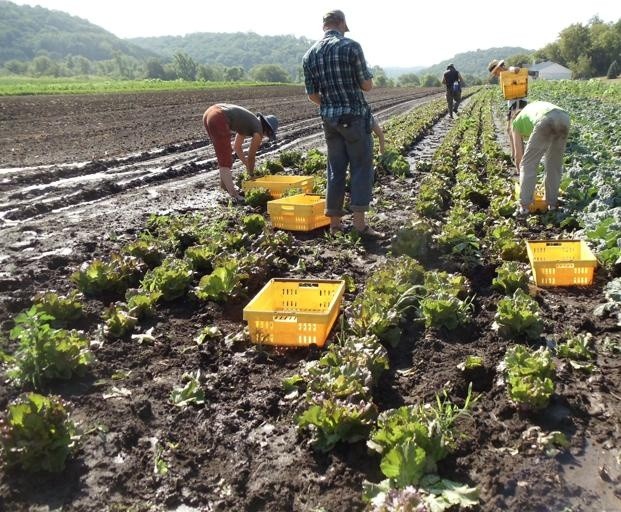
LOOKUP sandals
[330,226,348,238]
[358,225,385,240]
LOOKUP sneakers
[453,108,458,113]
[449,115,453,118]
[548,205,556,210]
[512,208,529,220]
[232,196,245,203]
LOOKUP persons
[302,9,386,239]
[488,60,528,142]
[510,101,570,219]
[374,121,384,155]
[203,103,278,201]
[442,63,466,119]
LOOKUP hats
[257,112,278,140]
[323,9,349,32]
[447,63,455,68]
[487,60,504,74]
